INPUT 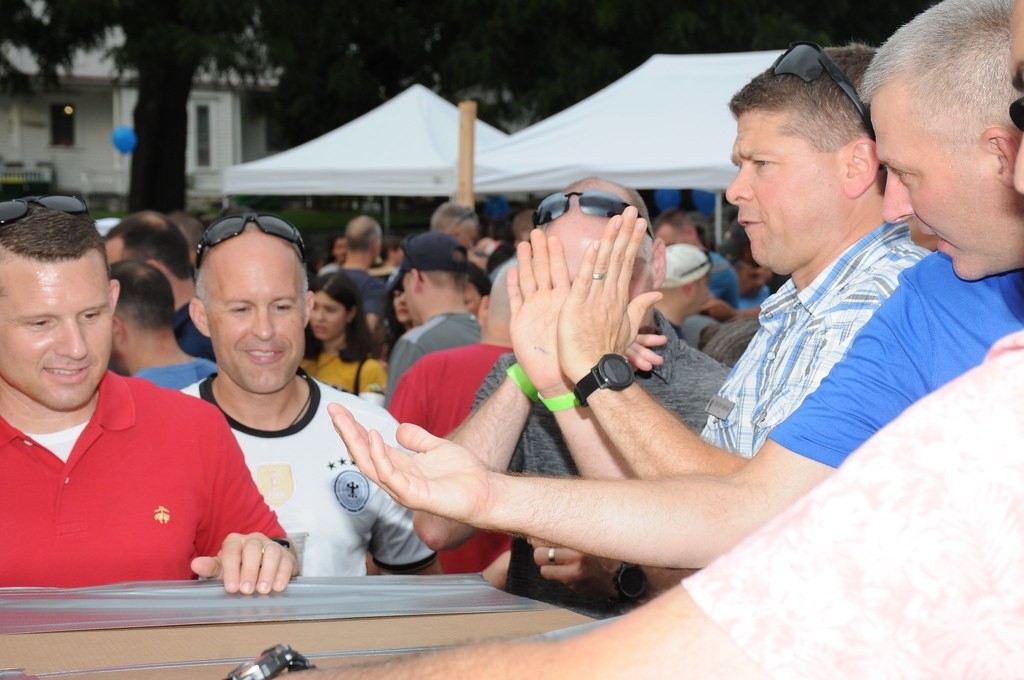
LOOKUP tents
[222,50,790,253]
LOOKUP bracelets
[537,390,580,412]
[506,363,539,403]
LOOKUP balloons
[111,126,137,155]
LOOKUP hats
[660,243,711,288]
[475,237,505,258]
[381,233,468,299]
[719,220,749,254]
[481,194,512,216]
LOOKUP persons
[327,0,1024,593]
[0,178,790,680]
[224,0,1024,680]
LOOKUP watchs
[610,562,647,603]
[224,643,318,680]
[272,538,290,548]
[574,354,636,408]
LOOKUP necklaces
[288,391,310,427]
[312,352,338,378]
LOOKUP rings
[262,541,283,555]
[548,547,555,565]
[592,273,606,280]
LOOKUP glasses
[452,206,478,231]
[470,247,489,264]
[0,193,90,225]
[681,249,714,277]
[721,246,761,269]
[196,213,306,270]
[1009,96,1024,132]
[531,189,656,242]
[773,41,876,143]
